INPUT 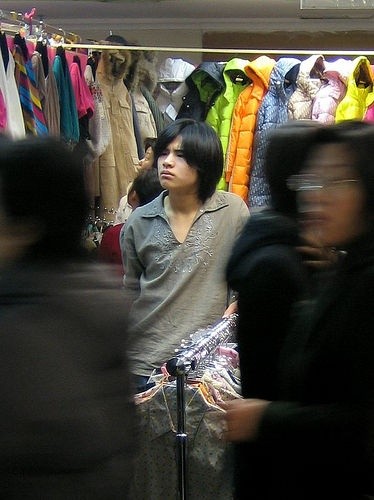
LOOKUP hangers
[0,7,372,426]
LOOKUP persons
[96,167,166,288]
[222,119,350,500]
[117,116,252,396]
[205,117,374,500]
[1,133,143,500]
[112,136,159,226]
